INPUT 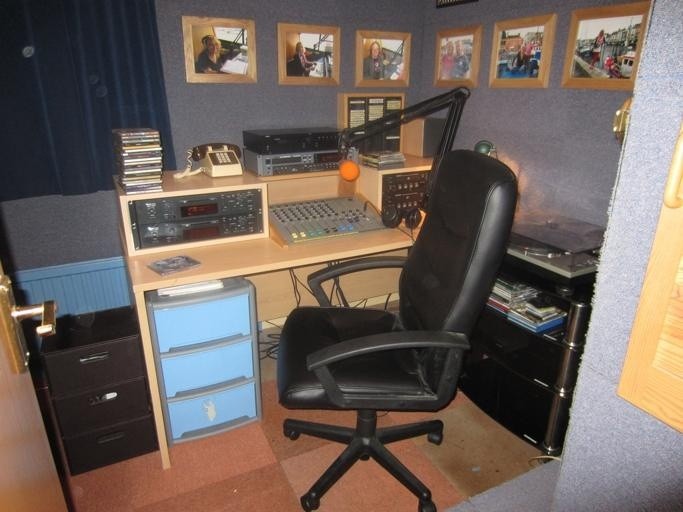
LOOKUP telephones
[191,143,243,178]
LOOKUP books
[111,127,163,195]
[486,277,566,334]
[361,150,406,167]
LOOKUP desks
[115,149,473,474]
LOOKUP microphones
[218,48,240,54]
[383,60,398,66]
[337,157,360,183]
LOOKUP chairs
[275,142,518,510]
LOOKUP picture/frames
[276,21,340,88]
[485,13,559,91]
[431,23,484,89]
[180,12,259,85]
[353,29,412,90]
[560,0,651,90]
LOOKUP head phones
[381,206,422,229]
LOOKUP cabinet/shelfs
[145,279,262,448]
[459,255,595,465]
[34,307,159,481]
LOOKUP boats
[577,41,594,57]
[603,54,634,79]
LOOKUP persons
[294,42,318,75]
[589,30,605,69]
[363,41,385,80]
[441,42,469,79]
[512,44,528,67]
[196,35,226,74]
